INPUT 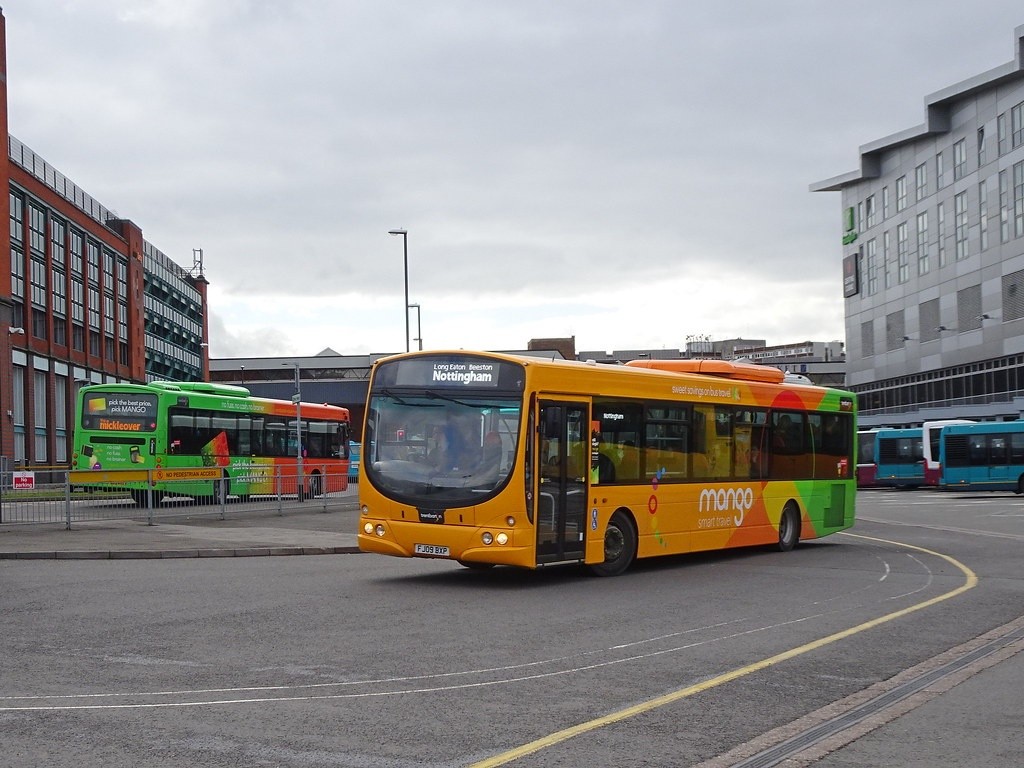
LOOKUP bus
[338,438,428,484]
[357,347,862,579]
[857,417,1024,496]
[67,379,352,507]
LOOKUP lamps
[9,326,25,337]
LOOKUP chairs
[482,432,503,473]
[789,423,822,449]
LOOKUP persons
[816,415,844,457]
[601,428,685,481]
[434,414,510,485]
[772,415,803,454]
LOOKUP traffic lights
[396,431,405,442]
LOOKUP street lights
[405,302,423,355]
[387,226,412,354]
